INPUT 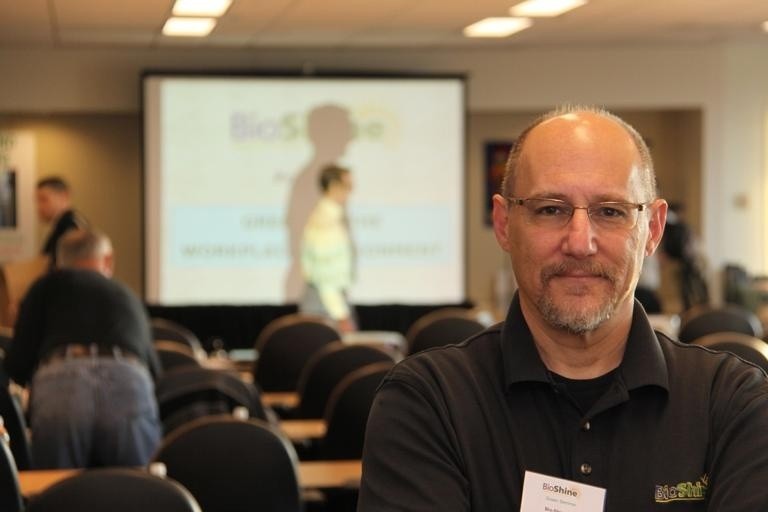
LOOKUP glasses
[499,188,659,236]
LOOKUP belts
[34,338,148,370]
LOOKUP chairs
[679,310,767,370]
[406,313,490,354]
[29,470,201,511]
[150,315,400,507]
[1,392,31,511]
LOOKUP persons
[8,226,166,470]
[353,103,767,510]
[722,281,759,318]
[298,161,361,338]
[32,176,85,272]
[718,263,749,290]
[660,197,709,315]
